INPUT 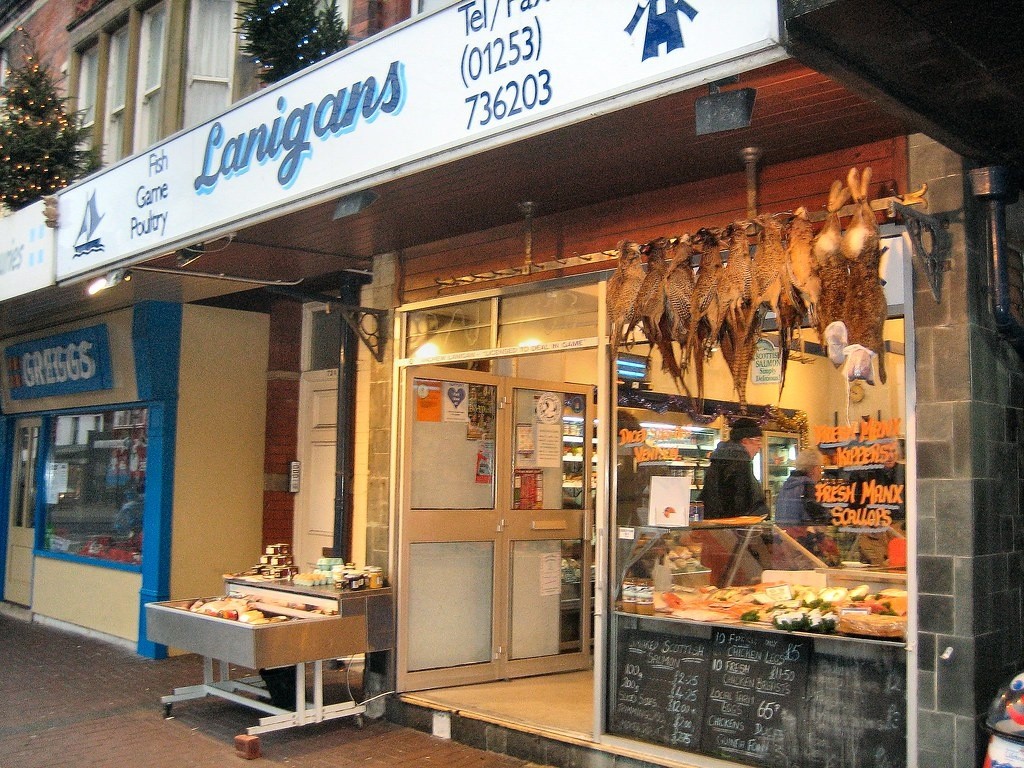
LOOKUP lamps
[104,266,132,288]
[175,242,206,267]
[694,75,758,136]
[332,188,381,220]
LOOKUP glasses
[746,437,762,444]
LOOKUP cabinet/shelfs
[561,403,803,644]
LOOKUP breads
[635,531,702,575]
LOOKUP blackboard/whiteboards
[610,611,907,768]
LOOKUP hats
[729,417,763,441]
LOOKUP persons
[593,410,672,578]
[850,437,905,519]
[775,449,832,528]
[702,417,772,587]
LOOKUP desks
[147,579,395,738]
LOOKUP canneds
[333,563,384,591]
[252,543,297,579]
[621,578,654,615]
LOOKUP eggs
[293,573,326,580]
